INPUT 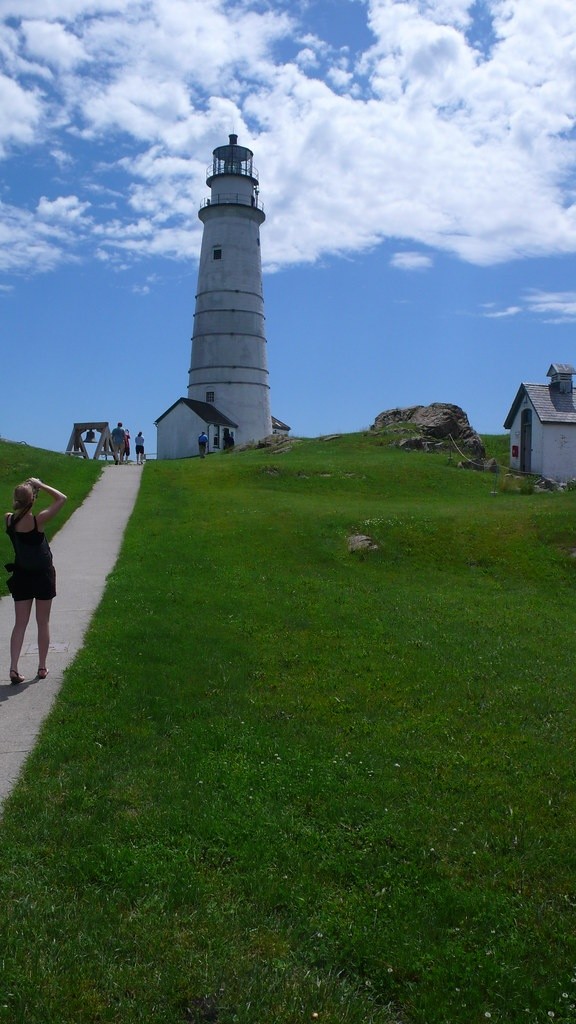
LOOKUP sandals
[38,668,49,679]
[10,670,24,685]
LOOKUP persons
[111,422,131,465]
[5,477,67,682]
[197,431,208,458]
[135,431,144,466]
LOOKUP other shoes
[119,462,124,465]
[137,463,140,465]
[115,461,118,465]
[141,462,143,464]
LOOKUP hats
[118,423,122,426]
